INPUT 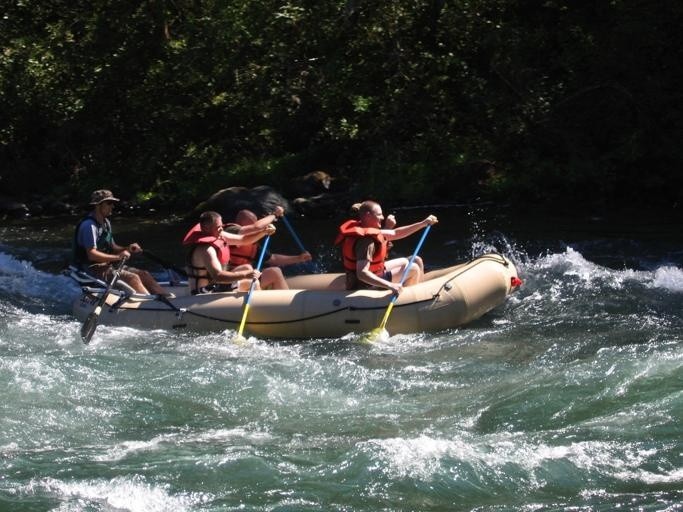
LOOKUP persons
[348,204,424,283]
[223,205,313,291]
[186,210,276,296]
[73,189,176,301]
[334,200,439,296]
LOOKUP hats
[87,189,120,205]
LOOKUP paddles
[372,217,432,336]
[80,254,124,342]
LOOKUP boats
[73,253,522,340]
